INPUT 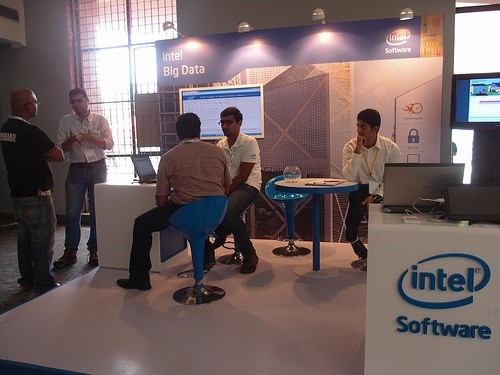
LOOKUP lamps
[312,8,326,25]
[163,21,185,37]
[237,22,254,33]
[400,7,414,21]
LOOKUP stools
[265,174,312,257]
[217,199,256,265]
[168,196,230,306]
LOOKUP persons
[340,108,402,270]
[187,106,264,273]
[53,88,114,269]
[0,88,66,291]
[116,112,233,289]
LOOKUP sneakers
[53,250,76,267]
[89,251,98,264]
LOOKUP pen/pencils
[324,180,340,182]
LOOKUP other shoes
[39,277,56,292]
[352,238,367,258]
[241,255,258,273]
[204,260,216,269]
[22,280,37,290]
[117,279,151,290]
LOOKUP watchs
[372,194,383,203]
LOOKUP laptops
[380,163,465,214]
[130,154,158,183]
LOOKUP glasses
[24,99,40,105]
[70,98,86,104]
[218,120,237,125]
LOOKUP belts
[38,189,51,195]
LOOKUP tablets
[442,184,500,225]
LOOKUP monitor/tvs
[179,84,265,139]
[450,72,500,131]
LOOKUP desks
[363,204,500,375]
[94,179,189,273]
[274,178,359,280]
